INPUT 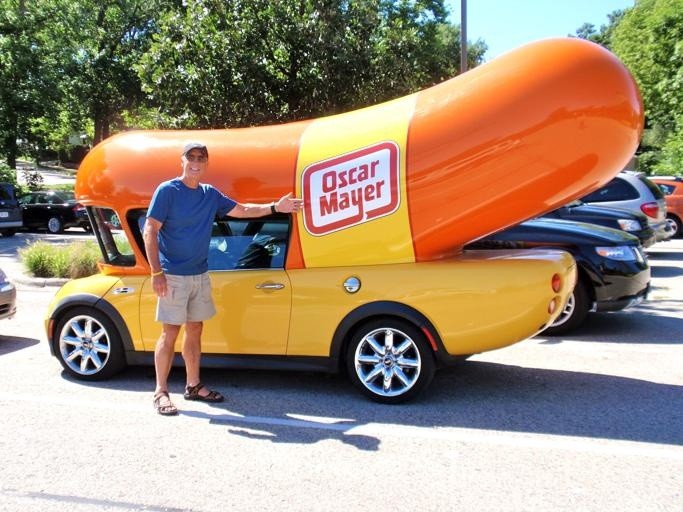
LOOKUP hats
[183,143,208,155]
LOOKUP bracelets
[151,271,163,276]
[271,203,277,215]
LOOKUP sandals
[153,391,178,415]
[183,382,223,402]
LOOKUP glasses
[184,154,206,162]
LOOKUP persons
[142,142,304,414]
